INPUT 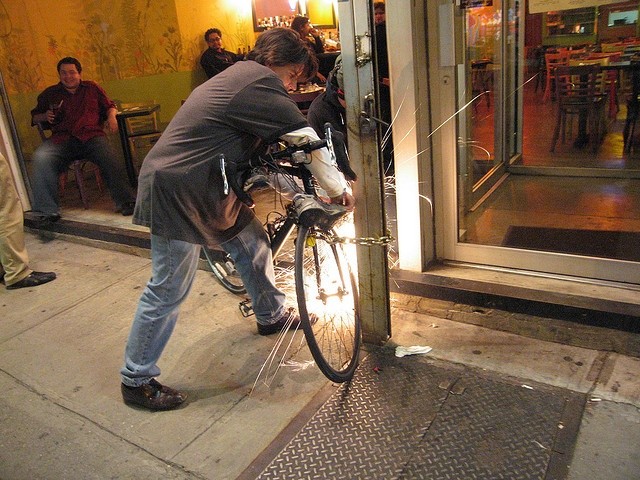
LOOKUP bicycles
[203,123,361,382]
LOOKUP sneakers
[291,193,346,231]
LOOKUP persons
[291,16,328,83]
[26,57,141,228]
[306,65,352,149]
[120,28,355,412]
[0,152,56,290]
[199,29,248,79]
[372,0,394,177]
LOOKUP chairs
[285,87,325,115]
[543,54,571,104]
[548,64,608,156]
[544,37,640,61]
[623,60,640,155]
[118,100,166,176]
[31,104,112,211]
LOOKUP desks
[113,103,160,193]
[548,60,639,150]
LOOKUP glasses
[209,37,221,42]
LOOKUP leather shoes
[121,203,134,216]
[6,271,57,290]
[256,309,319,336]
[49,212,60,222]
[120,378,188,409]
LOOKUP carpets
[502,224,639,264]
